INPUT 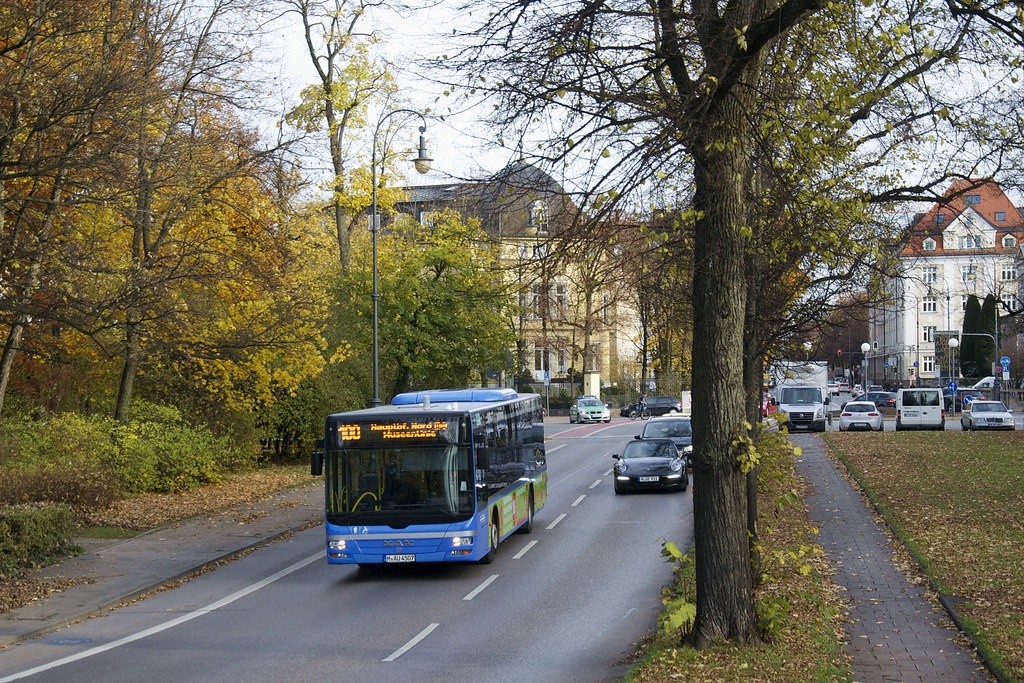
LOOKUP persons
[384,456,398,480]
[638,393,647,420]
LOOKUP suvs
[634,416,692,469]
[943,387,985,413]
[841,391,898,411]
[620,397,683,418]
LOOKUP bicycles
[629,402,653,420]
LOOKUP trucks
[775,360,830,433]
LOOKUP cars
[839,400,885,431]
[758,393,776,418]
[569,395,611,423]
[662,412,693,418]
[827,376,884,402]
[961,400,1016,431]
[612,438,690,494]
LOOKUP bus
[310,388,548,576]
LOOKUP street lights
[522,198,550,410]
[861,343,870,401]
[967,272,1001,401]
[845,325,851,369]
[948,338,959,417]
[368,109,435,408]
[900,292,921,387]
[923,274,951,376]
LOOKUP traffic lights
[873,341,878,352]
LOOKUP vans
[972,376,1015,389]
[894,388,945,431]
